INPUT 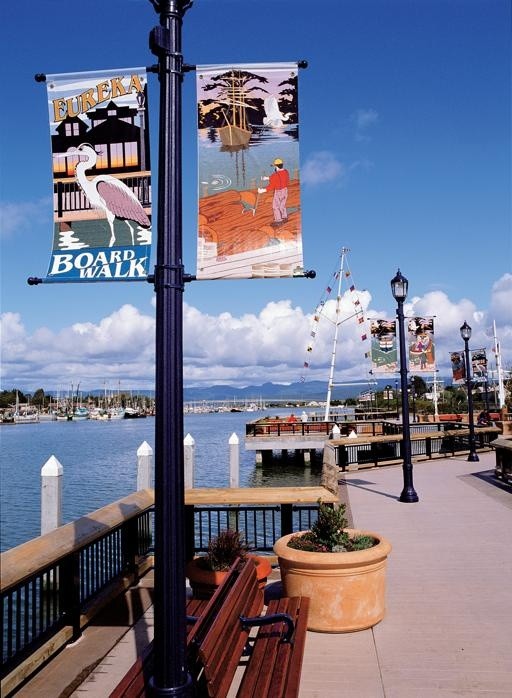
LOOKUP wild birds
[57,141,150,247]
[258,92,296,137]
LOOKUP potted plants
[188,529,271,600]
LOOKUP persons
[479,408,494,427]
[300,410,308,422]
[258,158,293,229]
[286,413,298,422]
[477,415,489,427]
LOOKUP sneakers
[270,222,283,227]
[282,218,288,223]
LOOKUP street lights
[386,268,419,503]
[459,320,480,462]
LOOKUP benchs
[198,558,312,698]
[109,556,242,698]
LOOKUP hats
[271,159,282,166]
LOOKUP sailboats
[217,70,252,147]
[0,379,268,424]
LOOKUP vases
[273,497,392,635]
